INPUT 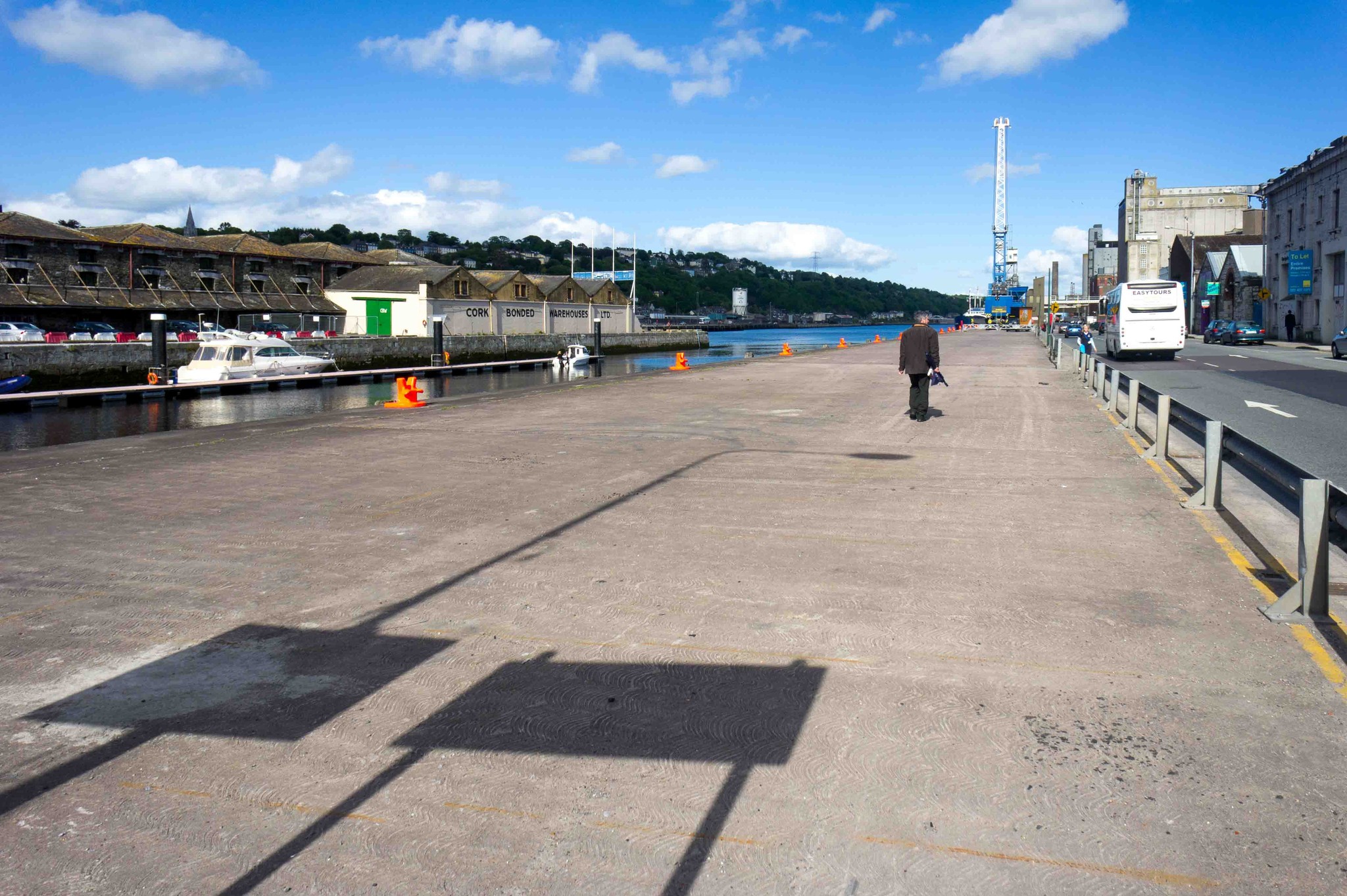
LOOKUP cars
[166,320,226,334]
[0,322,44,337]
[1331,326,1347,359]
[1204,320,1265,344]
[680,322,686,324]
[252,322,289,333]
[1089,321,1097,329]
[65,321,119,340]
[1065,324,1082,338]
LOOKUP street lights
[1165,226,1195,334]
[199,313,205,339]
[1146,241,1171,281]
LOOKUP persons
[1285,310,1296,342]
[898,311,940,422]
[1077,324,1097,372]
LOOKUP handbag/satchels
[1079,344,1085,354]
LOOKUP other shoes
[910,414,917,420]
[1078,367,1081,371]
[917,419,926,422]
[1086,369,1089,372]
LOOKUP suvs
[1099,322,1104,336]
[1058,323,1070,333]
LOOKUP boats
[174,331,336,384]
[0,374,33,394]
[552,333,591,367]
[955,285,985,330]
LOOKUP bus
[699,317,711,324]
[1103,279,1186,360]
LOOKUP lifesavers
[423,319,427,326]
[147,373,158,385]
